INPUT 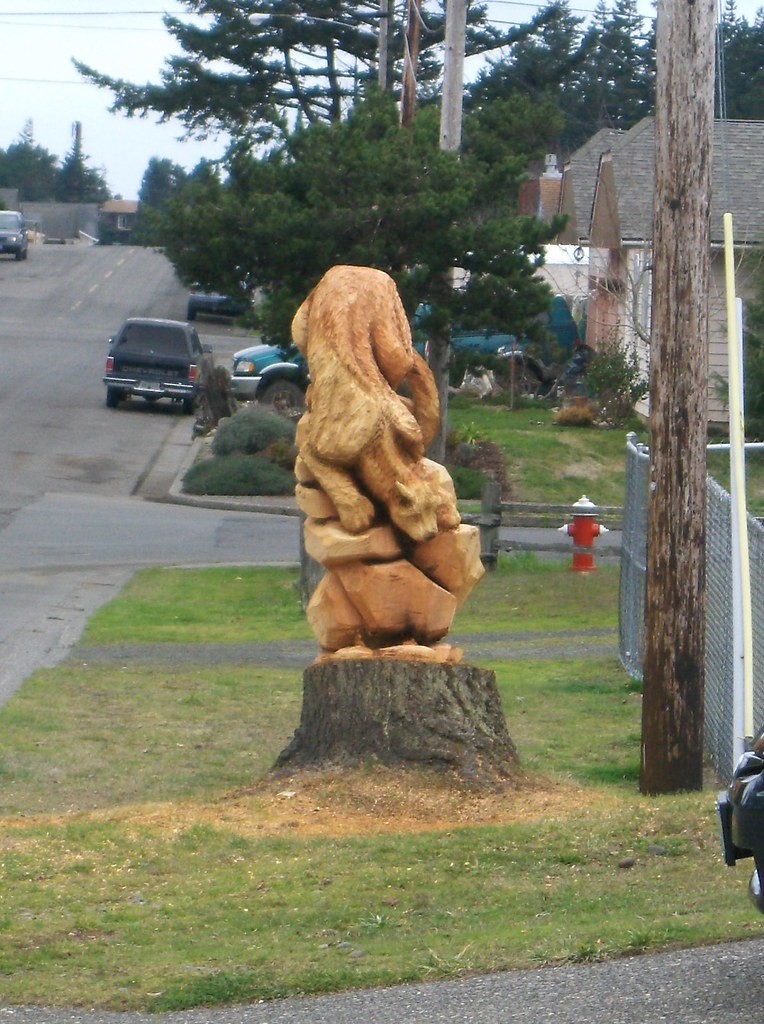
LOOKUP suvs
[0,210,28,263]
[100,317,215,417]
[231,290,583,410]
[186,266,251,323]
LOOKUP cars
[716,726,763,909]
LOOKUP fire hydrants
[559,494,609,573]
